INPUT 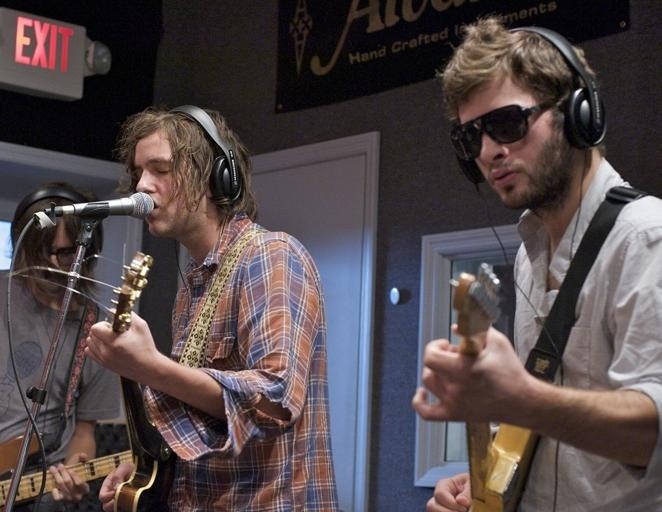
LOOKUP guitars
[448,262,539,512]
[0,431,136,512]
[110,251,177,512]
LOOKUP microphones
[45,192,156,220]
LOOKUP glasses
[45,246,78,268]
[449,93,567,163]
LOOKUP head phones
[445,27,607,184]
[166,103,246,207]
[10,188,103,250]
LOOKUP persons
[82,100,336,510]
[0,177,125,511]
[410,12,661,512]
